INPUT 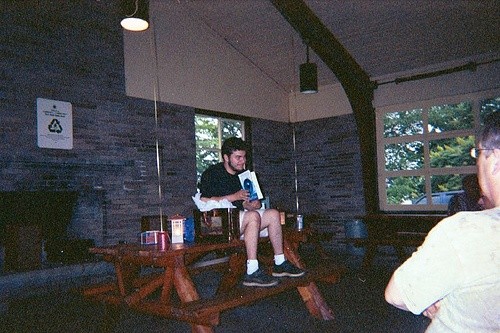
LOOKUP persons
[385,120,500,333]
[198,137,306,286]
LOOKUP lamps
[298,46,318,94]
[120,0,149,32]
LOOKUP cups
[298,214,302,231]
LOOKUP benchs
[64,255,231,299]
[349,238,423,248]
[180,261,345,317]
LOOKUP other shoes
[272,258,305,277]
[243,268,282,287]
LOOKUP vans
[413,190,465,205]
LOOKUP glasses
[470,146,494,159]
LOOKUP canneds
[157,231,169,252]
[294,214,303,231]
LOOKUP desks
[87,227,337,333]
[354,213,448,260]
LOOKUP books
[238,169,263,203]
